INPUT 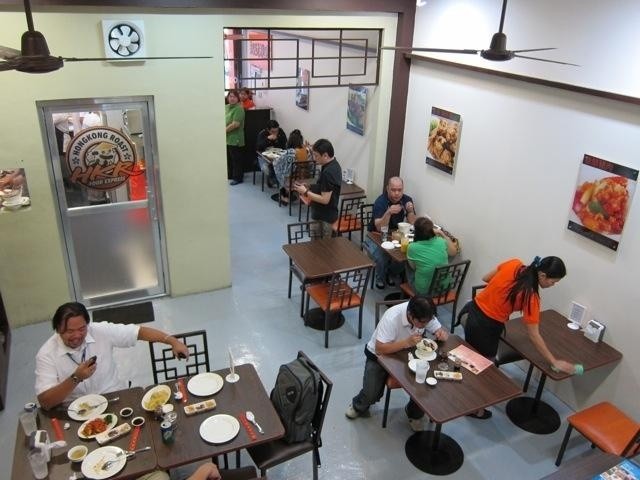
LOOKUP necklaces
[67,350,86,366]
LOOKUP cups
[20,413,38,437]
[401,238,409,253]
[381,226,388,241]
[27,447,49,480]
[415,360,428,384]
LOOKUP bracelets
[163,333,169,342]
[304,188,309,197]
[70,372,83,385]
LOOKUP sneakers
[375,277,386,291]
[387,275,396,289]
[405,404,425,433]
[344,400,360,419]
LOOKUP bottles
[454,358,462,371]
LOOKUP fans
[1,1,214,75]
[382,0,582,68]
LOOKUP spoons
[245,411,265,433]
[174,383,183,400]
[58,408,87,414]
[101,452,136,470]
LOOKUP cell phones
[89,355,97,367]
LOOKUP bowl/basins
[398,223,411,233]
[141,385,171,411]
[416,338,438,356]
[67,445,89,463]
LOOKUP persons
[134,462,223,480]
[362,175,417,290]
[30,300,191,412]
[294,137,344,291]
[256,118,289,189]
[344,294,450,434]
[404,217,459,300]
[237,87,256,109]
[464,253,580,420]
[226,89,247,186]
[278,128,311,206]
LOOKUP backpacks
[270,356,324,445]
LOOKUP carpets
[91,302,157,327]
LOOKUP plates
[405,234,414,242]
[184,405,194,415]
[81,446,127,480]
[380,241,394,249]
[132,416,145,427]
[67,394,108,421]
[392,239,400,244]
[426,377,438,385]
[77,413,118,440]
[415,349,437,361]
[187,372,224,397]
[408,359,431,373]
[194,401,206,412]
[395,244,401,248]
[204,398,217,410]
[120,407,134,417]
[261,147,287,158]
[199,413,240,444]
[96,423,131,445]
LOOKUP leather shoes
[467,408,492,420]
[230,179,240,185]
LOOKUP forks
[116,446,153,457]
[88,397,119,409]
